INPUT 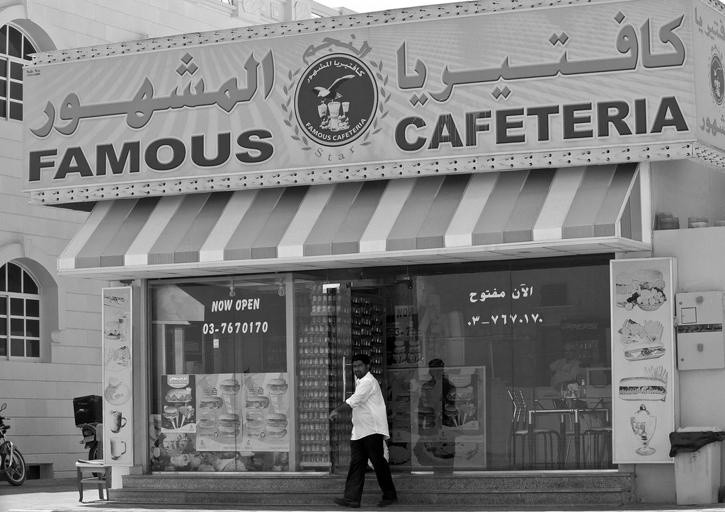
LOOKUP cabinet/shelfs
[297,293,387,470]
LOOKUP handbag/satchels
[367,439,390,471]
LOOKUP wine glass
[631,416,657,456]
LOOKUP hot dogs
[620,377,667,401]
[623,344,666,361]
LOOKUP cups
[111,411,127,433]
[110,437,126,460]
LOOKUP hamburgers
[197,378,288,439]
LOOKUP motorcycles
[76,422,102,477]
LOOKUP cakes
[434,373,482,460]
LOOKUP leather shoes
[333,495,361,509]
[377,495,398,507]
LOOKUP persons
[417,357,460,474]
[326,352,399,508]
[544,341,590,387]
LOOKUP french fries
[644,364,668,382]
[642,320,663,338]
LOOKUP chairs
[75,424,112,502]
[506,383,612,468]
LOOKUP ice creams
[631,404,656,442]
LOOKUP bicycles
[0,403,26,486]
